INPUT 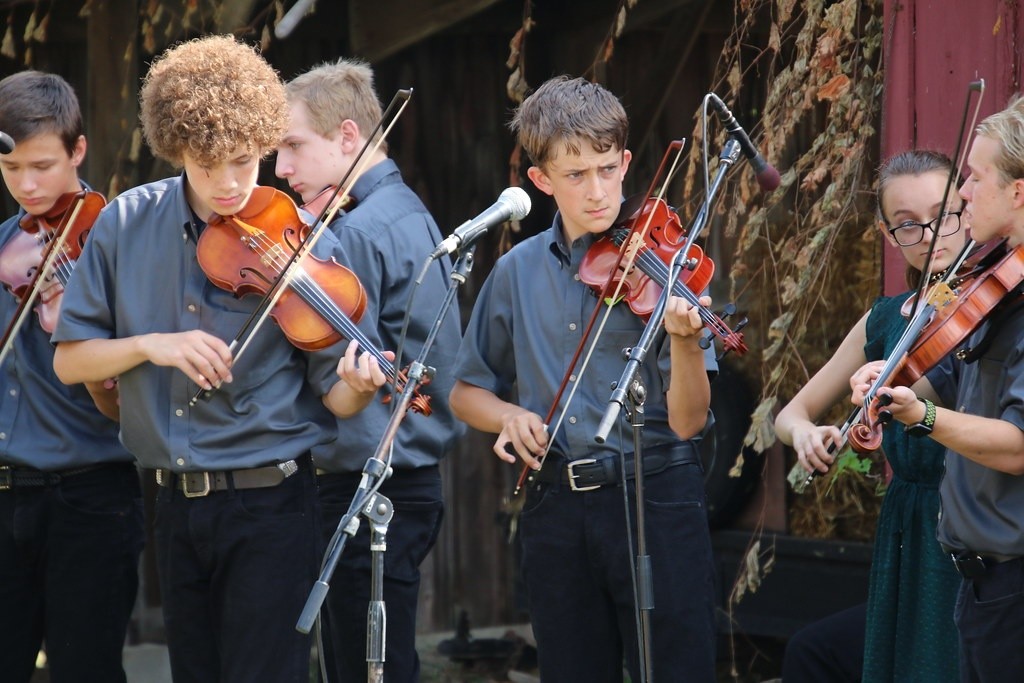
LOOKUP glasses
[885,202,967,247]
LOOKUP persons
[0,33,462,683]
[776,103,1024,683]
[448,74,723,683]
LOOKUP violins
[194,185,434,418]
[297,185,355,221]
[844,232,1023,457]
[579,196,750,361]
[1,188,123,413]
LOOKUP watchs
[904,397,936,439]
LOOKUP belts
[0,466,108,491]
[526,441,699,492]
[950,553,999,581]
[155,450,311,499]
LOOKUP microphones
[709,93,780,191]
[0,131,15,154]
[430,187,532,262]
